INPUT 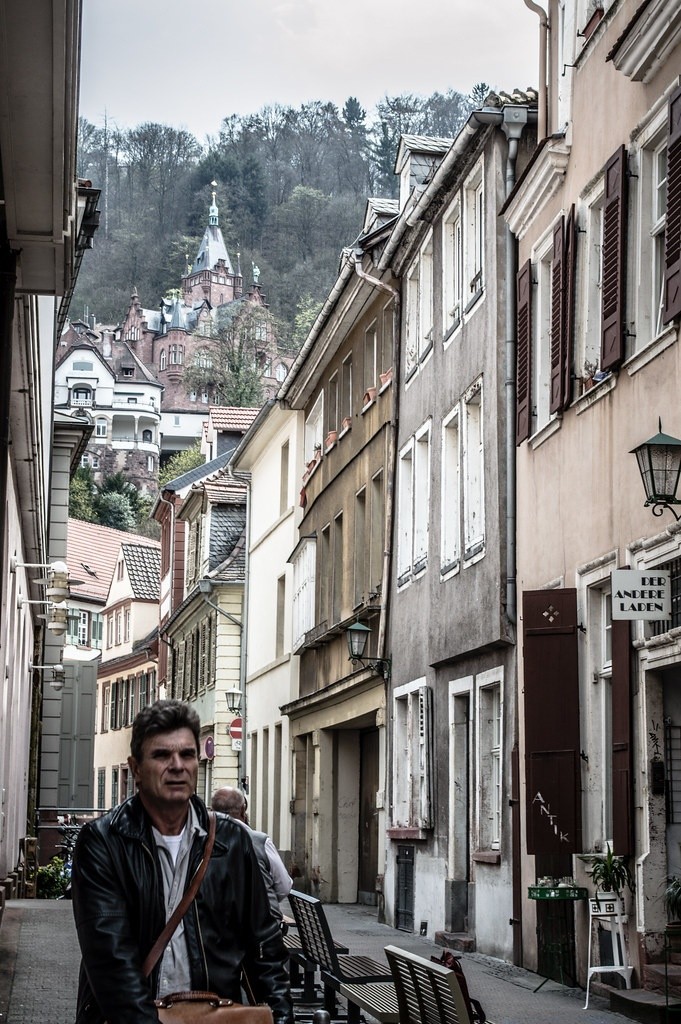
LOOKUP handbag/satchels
[151,991,275,1023]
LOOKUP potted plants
[583,837,637,912]
[652,869,681,941]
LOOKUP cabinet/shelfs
[582,896,634,1010]
[662,930,681,1024]
[527,886,586,995]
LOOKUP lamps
[343,621,392,679]
[225,681,243,717]
[626,415,681,522]
[5,553,70,692]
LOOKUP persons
[212,787,293,936]
[71,699,294,1024]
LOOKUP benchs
[276,884,493,1024]
